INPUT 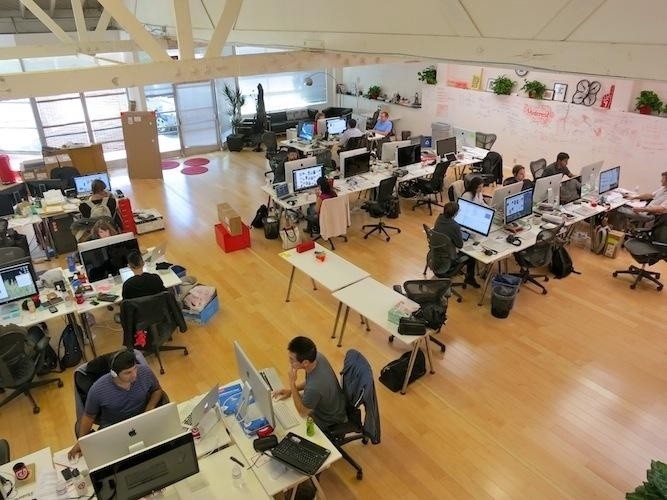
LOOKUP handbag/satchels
[378,349,426,393]
[398,317,427,335]
[279,226,302,250]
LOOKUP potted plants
[367,86,381,99]
[416,65,437,85]
[634,90,663,115]
[220,83,245,151]
[491,75,517,95]
[521,80,544,99]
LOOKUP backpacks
[551,246,582,279]
[58,323,81,368]
[251,205,267,229]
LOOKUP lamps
[304,71,341,107]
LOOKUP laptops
[255,431,331,478]
[180,382,225,429]
[444,152,463,164]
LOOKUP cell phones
[60,467,73,481]
[48,306,58,313]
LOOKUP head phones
[481,245,498,256]
[506,234,521,246]
[110,351,127,378]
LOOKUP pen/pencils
[230,457,244,467]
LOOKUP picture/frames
[542,89,555,100]
[553,83,568,102]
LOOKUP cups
[533,215,542,225]
[73,475,88,496]
[13,462,29,480]
[592,201,598,207]
[67,256,76,272]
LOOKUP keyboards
[96,293,119,302]
[41,296,64,308]
[272,402,301,430]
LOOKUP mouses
[70,451,79,464]
[273,393,284,402]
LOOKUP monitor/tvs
[0,262,39,314]
[381,140,411,163]
[317,117,340,138]
[351,113,367,133]
[284,156,317,191]
[581,160,603,191]
[559,175,581,205]
[25,179,68,201]
[398,144,421,170]
[533,173,563,203]
[326,117,347,135]
[77,232,134,265]
[81,237,143,283]
[491,181,524,209]
[89,430,200,500]
[0,182,28,217]
[292,163,324,192]
[339,147,367,175]
[453,197,495,237]
[344,152,370,178]
[504,188,533,225]
[72,170,112,198]
[599,166,620,194]
[233,340,276,430]
[296,119,315,145]
[78,401,184,471]
[436,136,456,162]
[0,257,38,281]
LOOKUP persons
[90,179,114,201]
[434,202,481,289]
[270,336,349,429]
[122,250,167,299]
[77,219,118,265]
[461,176,489,208]
[506,153,667,228]
[68,350,162,459]
[274,108,393,234]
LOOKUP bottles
[232,463,241,488]
[55,477,69,500]
[27,297,38,317]
[108,274,115,289]
[60,289,72,307]
[306,417,314,436]
[635,185,639,195]
[553,199,557,212]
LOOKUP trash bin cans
[262,216,280,239]
[421,136,431,147]
[171,265,186,294]
[491,285,517,319]
[402,131,411,141]
[491,273,521,309]
[178,275,200,295]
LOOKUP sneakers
[464,277,481,288]
